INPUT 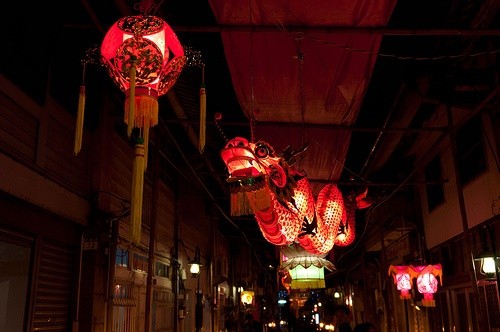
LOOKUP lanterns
[389,264,443,307]
[73,13,207,245]
[220,137,370,291]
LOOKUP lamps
[180,239,201,278]
[334,287,340,299]
[472,226,496,276]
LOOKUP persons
[241,304,376,332]
